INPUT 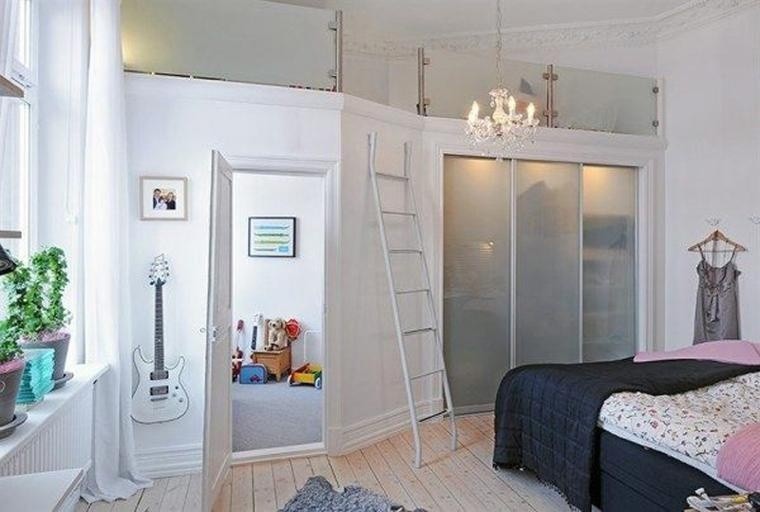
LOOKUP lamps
[461,0,542,165]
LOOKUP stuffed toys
[264,318,287,351]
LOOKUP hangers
[688,229,745,253]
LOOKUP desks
[0,467,87,512]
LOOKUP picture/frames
[137,175,189,221]
[248,216,297,257]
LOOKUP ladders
[368,130,457,468]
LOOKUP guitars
[244,312,266,365]
[129,254,188,424]
[231,319,244,381]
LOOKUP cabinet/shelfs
[251,348,290,382]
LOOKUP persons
[152,188,175,209]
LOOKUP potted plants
[1,266,28,440]
[15,245,75,393]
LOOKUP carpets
[275,473,435,512]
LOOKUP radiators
[0,383,94,479]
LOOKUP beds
[490,338,760,512]
